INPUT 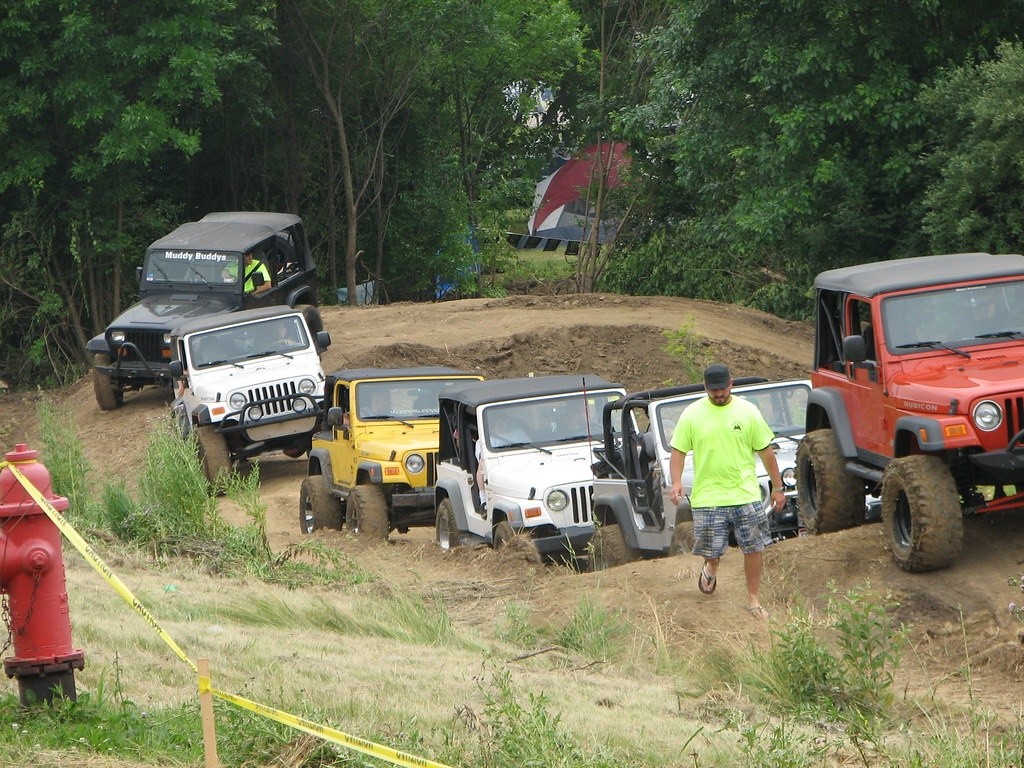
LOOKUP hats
[704,365,731,390]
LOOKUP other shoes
[480,502,488,520]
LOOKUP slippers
[744,600,770,622]
[698,557,720,594]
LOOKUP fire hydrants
[0,444,86,710]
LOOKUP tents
[527,139,658,237]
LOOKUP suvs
[586,375,814,569]
[432,375,644,570]
[166,305,331,499]
[296,366,486,544]
[792,252,1024,575]
[85,211,322,411]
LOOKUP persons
[669,363,786,619]
[222,247,272,296]
[266,320,296,350]
[177,337,229,398]
[453,401,595,518]
[342,387,392,425]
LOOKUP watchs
[772,487,784,493]
